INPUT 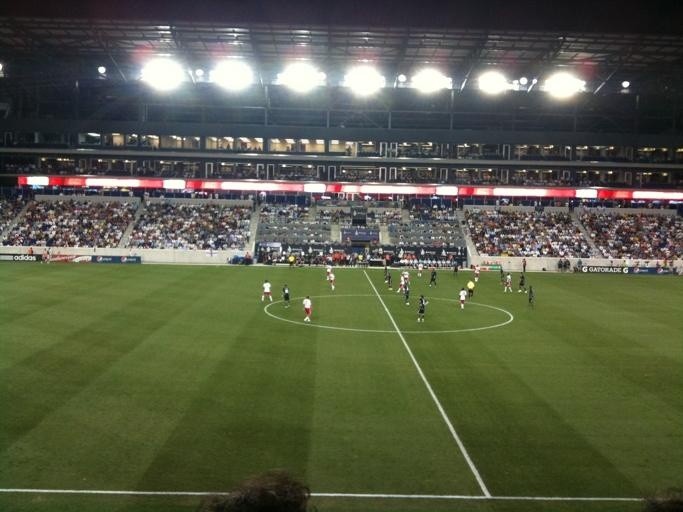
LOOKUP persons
[190,471,313,511]
[642,484,683,510]
